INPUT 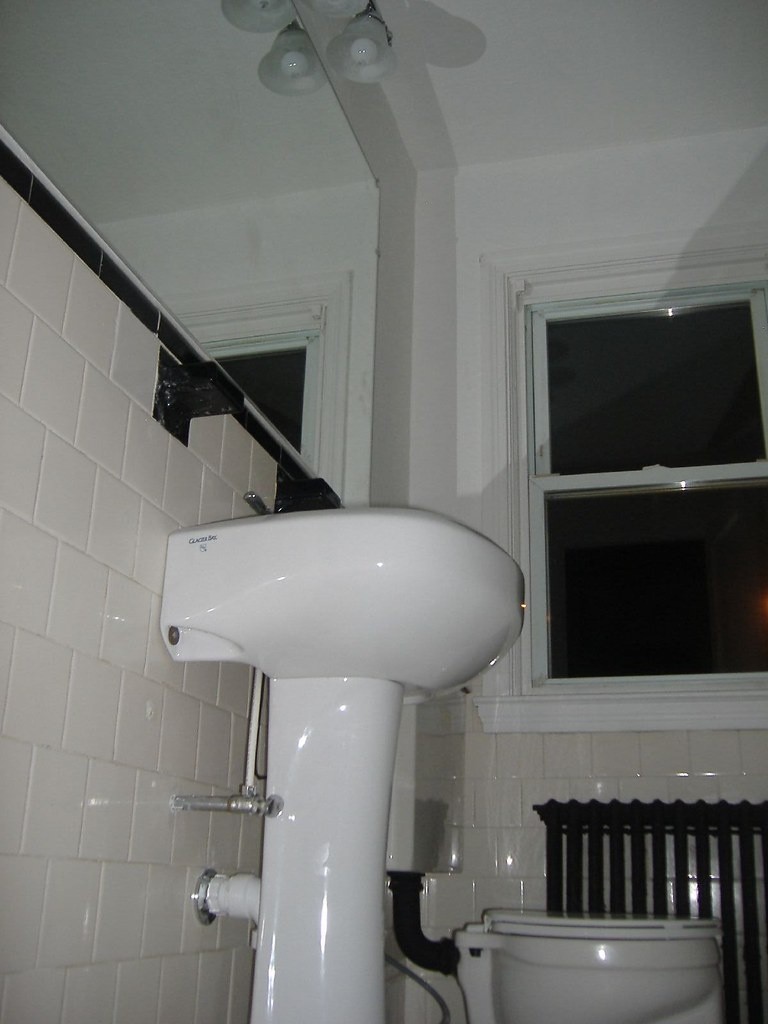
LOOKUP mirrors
[0,0,380,510]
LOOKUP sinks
[157,505,527,707]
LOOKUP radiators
[533,797,768,1024]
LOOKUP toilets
[387,688,729,1024]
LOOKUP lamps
[221,0,328,97]
[301,0,395,83]
